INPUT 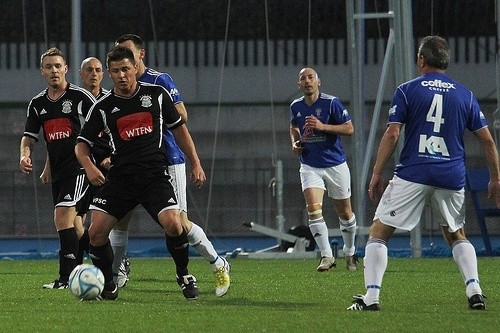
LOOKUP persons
[344,37,500,311]
[290,67,359,271]
[109,34,231,297]
[20,47,129,288]
[40,57,131,276]
[75,44,199,301]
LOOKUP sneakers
[117,259,131,287]
[94,285,119,301]
[345,253,359,271]
[212,256,231,296]
[317,256,336,271]
[468,294,488,310]
[176,275,198,300]
[346,294,380,312]
[43,280,70,289]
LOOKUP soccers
[69,264,105,299]
